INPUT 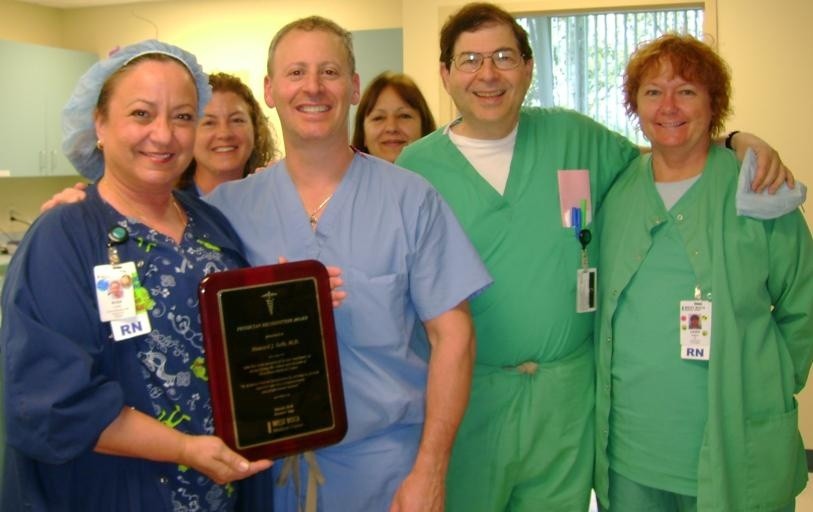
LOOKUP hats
[59,39,213,181]
[734,148,805,221]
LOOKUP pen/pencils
[582,200,586,230]
[577,208,581,239]
[572,207,576,227]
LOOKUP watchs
[724,129,738,148]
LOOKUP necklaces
[311,194,334,225]
[100,178,187,240]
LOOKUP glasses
[450,47,523,73]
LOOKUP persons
[588,33,813,512]
[349,68,438,164]
[0,38,350,512]
[394,1,797,512]
[175,67,275,202]
[40,14,492,511]
